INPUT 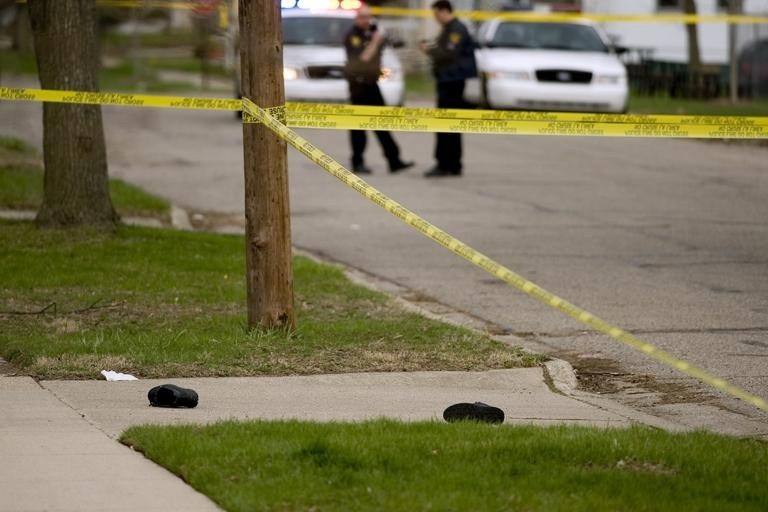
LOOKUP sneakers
[146,383,199,409]
[442,400,506,426]
[355,159,463,178]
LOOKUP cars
[463,16,643,115]
[233,1,406,119]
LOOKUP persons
[420,1,478,176]
[343,7,415,174]
